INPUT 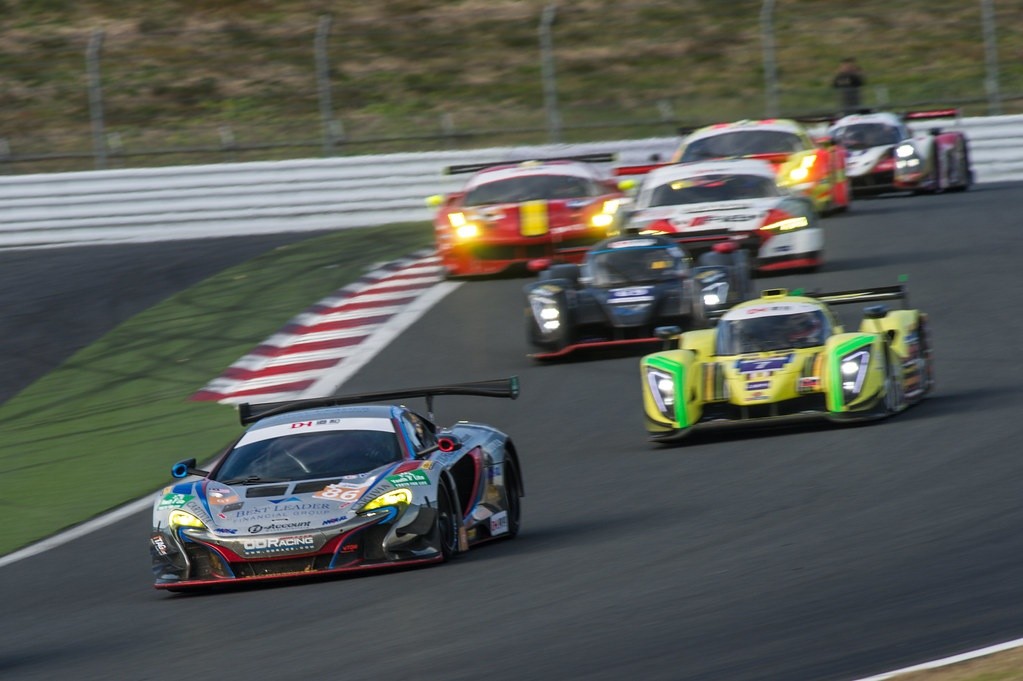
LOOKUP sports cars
[522,231,754,362]
[423,149,639,279]
[670,112,849,216]
[609,150,827,274]
[147,374,528,593]
[639,282,934,449]
[812,106,974,199]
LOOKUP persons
[832,58,865,109]
